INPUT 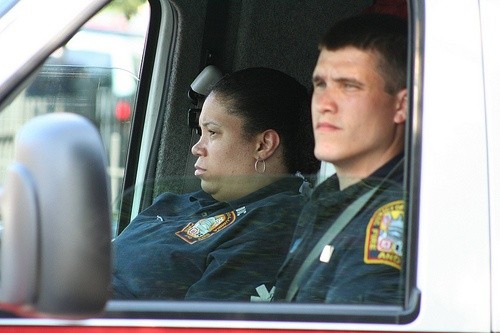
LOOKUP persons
[267,19,411,313]
[105,65,316,305]
